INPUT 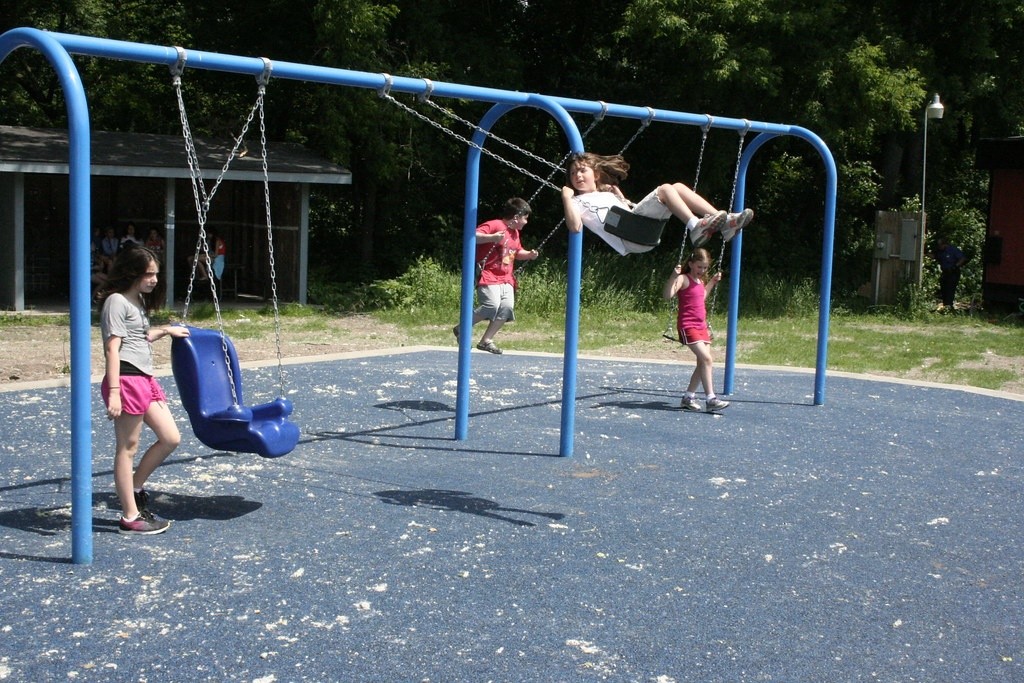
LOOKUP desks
[218,264,245,301]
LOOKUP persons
[144,227,164,272]
[101,226,119,263]
[925,238,969,306]
[560,151,754,257]
[187,225,227,281]
[92,226,113,275]
[92,239,109,305]
[119,221,145,249]
[453,198,539,354]
[100,236,191,536]
[663,248,730,412]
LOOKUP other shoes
[198,276,208,280]
[477,339,503,354]
[453,326,461,344]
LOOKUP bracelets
[109,387,121,389]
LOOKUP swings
[663,133,745,343]
[477,123,650,286]
[386,93,669,246]
[170,71,300,459]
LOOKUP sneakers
[118,511,171,534]
[680,396,702,411]
[721,208,755,242]
[689,209,728,247]
[133,489,154,520]
[706,397,730,412]
